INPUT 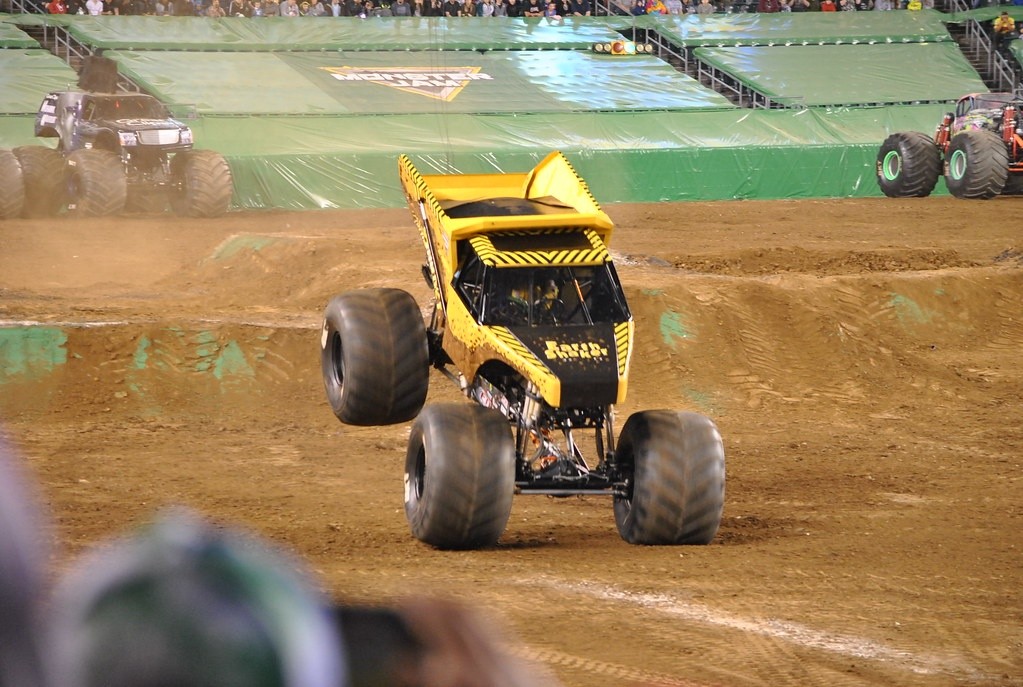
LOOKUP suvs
[320,148,725,551]
[876,92,1023,199]
[12,46,233,219]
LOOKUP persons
[511,278,562,316]
[34,514,560,687]
[13,0,934,19]
[992,12,1015,52]
